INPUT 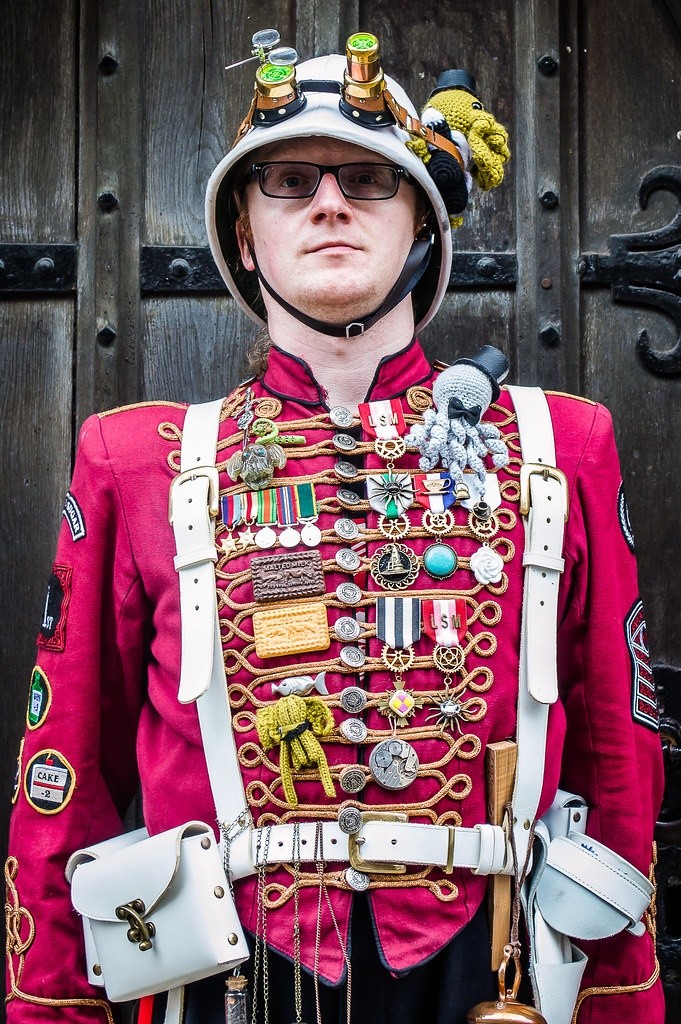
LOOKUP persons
[0,25,672,1024]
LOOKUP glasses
[241,156,414,200]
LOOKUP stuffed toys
[395,342,514,484]
[402,63,516,237]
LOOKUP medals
[226,379,510,796]
[250,693,340,810]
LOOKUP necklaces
[132,802,560,1024]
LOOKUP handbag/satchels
[64,821,251,1003]
[64,827,150,986]
[540,785,586,840]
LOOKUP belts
[223,820,537,877]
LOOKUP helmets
[203,55,453,330]
